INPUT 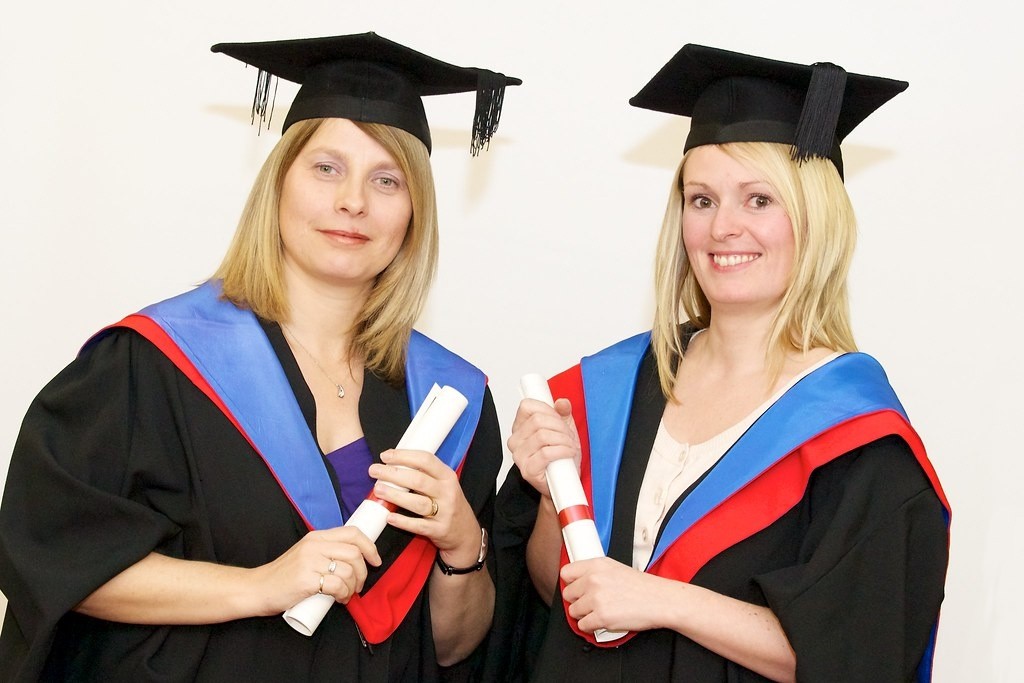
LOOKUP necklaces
[280,319,345,399]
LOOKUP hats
[211,32,523,156]
[629,43,909,182]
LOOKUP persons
[0,33,503,683]
[495,43,952,683]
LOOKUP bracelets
[428,528,488,576]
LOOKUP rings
[329,561,336,573]
[318,575,323,594]
[423,496,438,516]
